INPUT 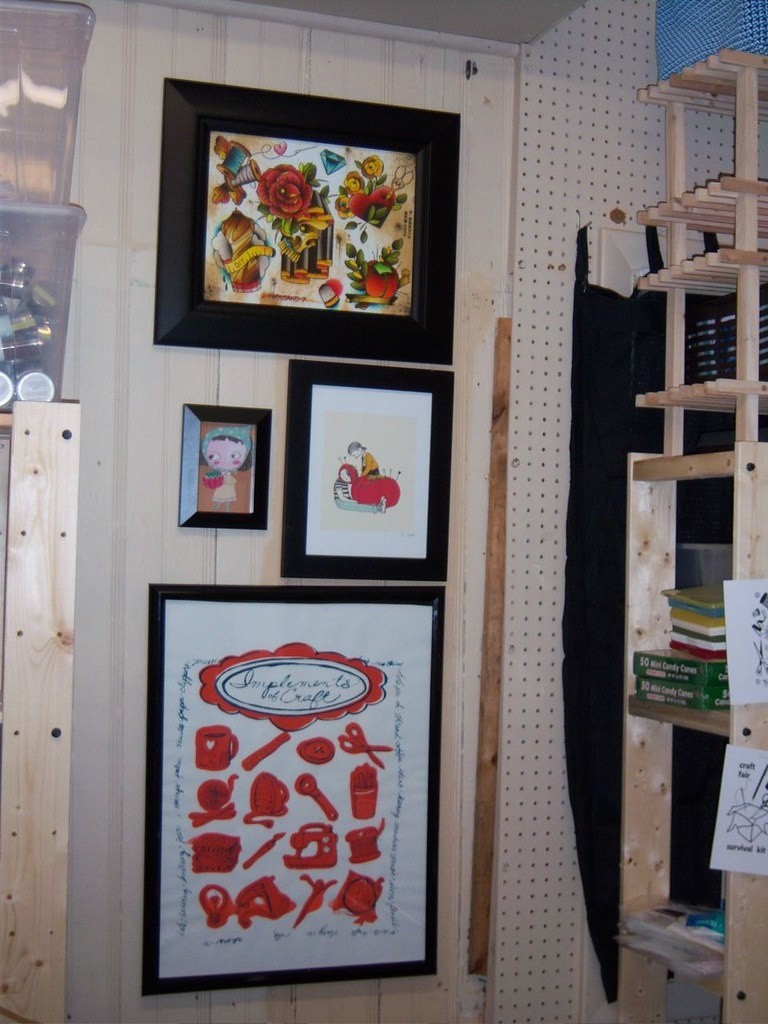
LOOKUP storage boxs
[634,651,729,684]
[0,203,88,411]
[636,678,731,707]
[0,0,95,206]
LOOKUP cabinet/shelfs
[584,50,763,1024]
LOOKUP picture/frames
[280,356,455,580]
[176,404,274,533]
[137,583,440,995]
[150,73,465,367]
